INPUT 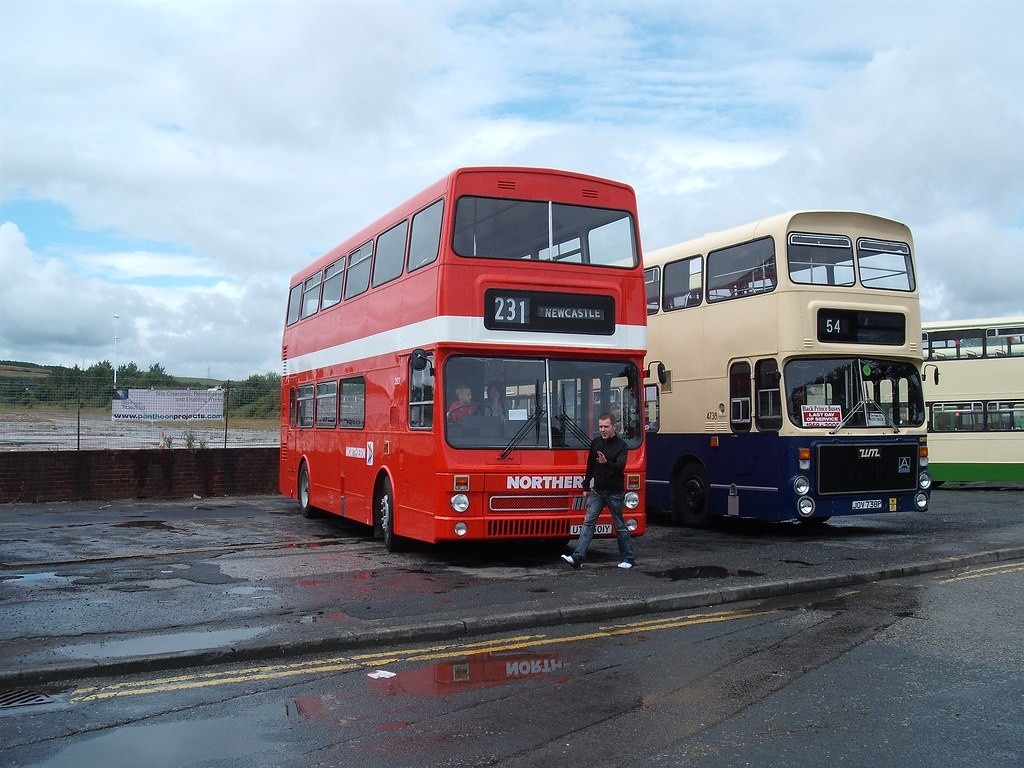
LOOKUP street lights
[111,315,120,390]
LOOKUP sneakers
[561,554,577,566]
[618,562,632,568]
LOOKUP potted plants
[181,429,197,447]
[164,435,174,448]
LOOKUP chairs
[684,290,701,307]
[966,350,977,358]
[995,349,1007,357]
[935,352,947,360]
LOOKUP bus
[276,168,666,555]
[480,207,940,533]
[822,314,1024,490]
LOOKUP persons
[561,412,636,569]
[447,384,481,423]
[484,385,512,417]
